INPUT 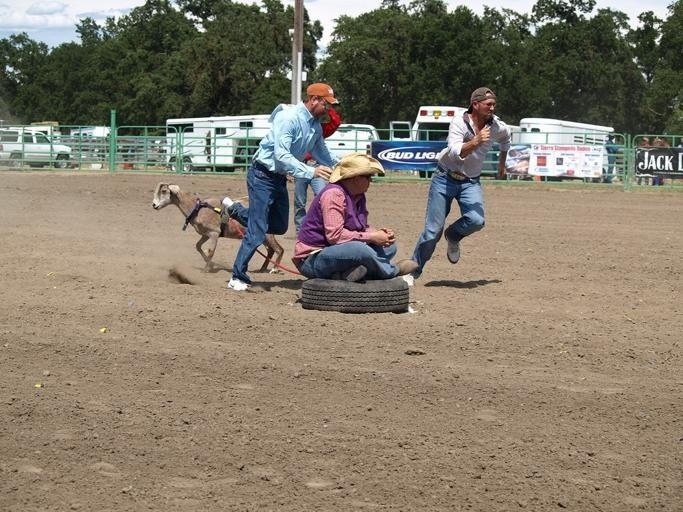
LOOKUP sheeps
[151,181,284,274]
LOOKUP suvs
[0,128,72,169]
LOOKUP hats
[306,83,338,105]
[329,153,385,185]
[465,87,497,113]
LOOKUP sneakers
[228,277,252,292]
[395,259,420,277]
[404,274,416,289]
[444,227,461,264]
[332,266,368,283]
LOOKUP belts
[436,164,483,185]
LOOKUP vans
[387,104,505,179]
[321,123,382,176]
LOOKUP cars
[61,137,160,167]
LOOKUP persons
[221,82,340,292]
[402,86,511,285]
[291,153,419,282]
[286,99,341,236]
[605,131,617,183]
[636,136,682,187]
